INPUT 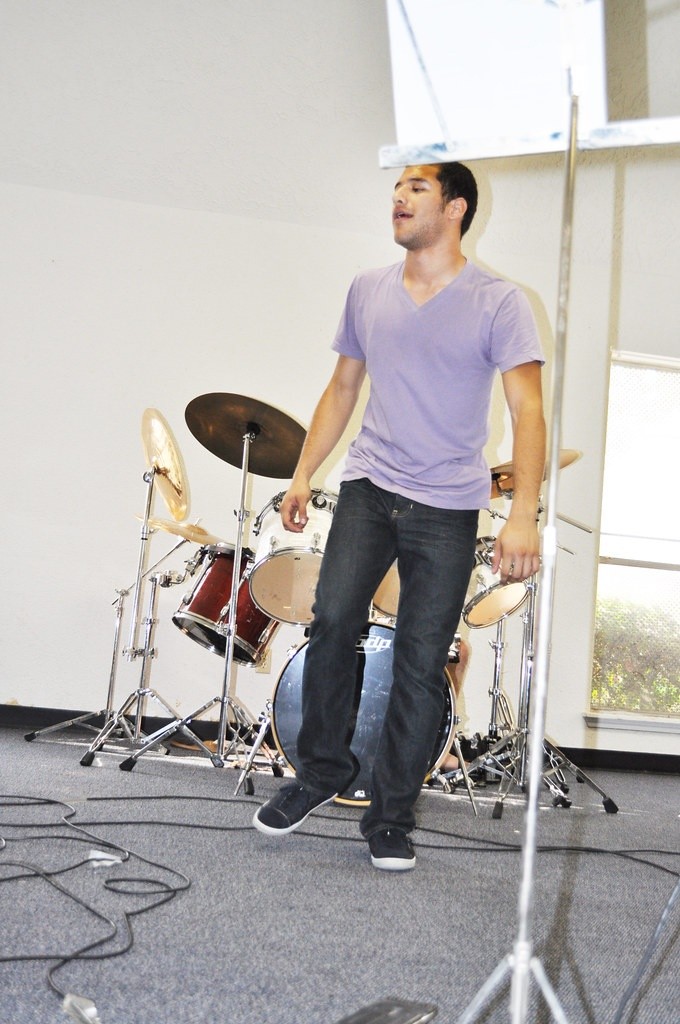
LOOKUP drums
[249,486,343,626]
[372,556,402,619]
[166,544,282,668]
[461,535,538,627]
[269,614,463,808]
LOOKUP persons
[251,159,547,870]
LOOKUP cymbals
[184,391,308,480]
[138,405,190,516]
[485,449,584,500]
[144,516,225,545]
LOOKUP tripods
[24,433,284,794]
[428,565,620,820]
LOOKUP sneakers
[252,783,339,836]
[368,828,416,871]
